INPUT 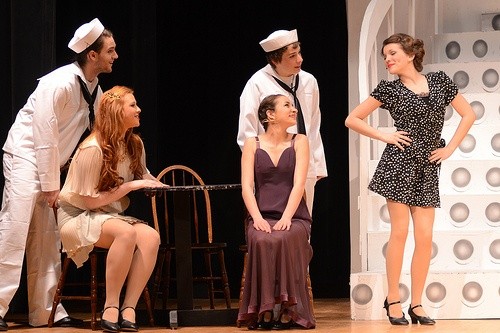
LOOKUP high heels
[256,308,274,330]
[118,306,138,332]
[273,311,293,330]
[408,304,436,325]
[383,296,409,325]
[100,306,121,333]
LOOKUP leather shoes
[0,315,7,330]
[41,316,85,327]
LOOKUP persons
[345,32,477,325]
[0,18,118,331]
[236,29,328,330]
[57,86,170,333]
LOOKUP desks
[141,184,243,329]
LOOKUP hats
[258,29,298,52]
[68,17,105,54]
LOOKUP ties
[272,74,306,136]
[77,75,99,131]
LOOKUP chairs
[49,164,154,330]
[150,164,232,309]
